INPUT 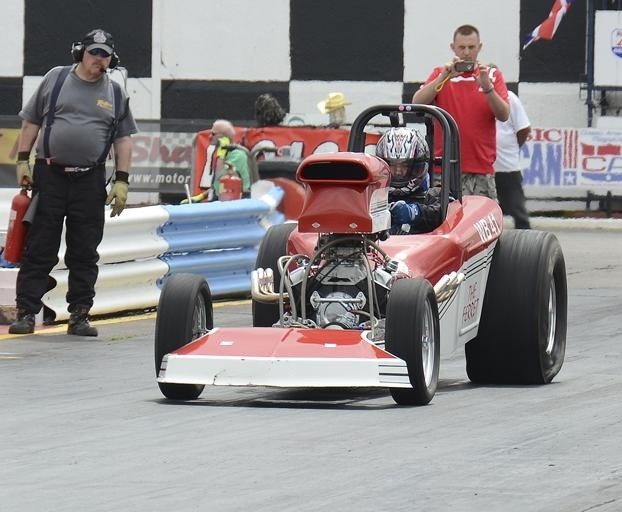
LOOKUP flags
[522,0,575,50]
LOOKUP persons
[376,127,453,235]
[483,59,532,228]
[180,119,250,204]
[322,92,347,124]
[5,28,138,336]
[412,24,510,210]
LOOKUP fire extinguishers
[3,176,33,263]
[218,162,242,201]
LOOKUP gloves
[16,152,34,187]
[105,171,129,217]
[387,199,422,226]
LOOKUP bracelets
[482,83,495,94]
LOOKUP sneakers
[8,307,35,333]
[67,304,98,336]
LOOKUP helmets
[375,127,430,198]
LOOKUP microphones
[100,65,117,74]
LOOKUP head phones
[71,42,119,69]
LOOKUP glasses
[88,49,110,58]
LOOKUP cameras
[455,61,474,72]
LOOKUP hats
[81,28,115,55]
[324,93,352,114]
